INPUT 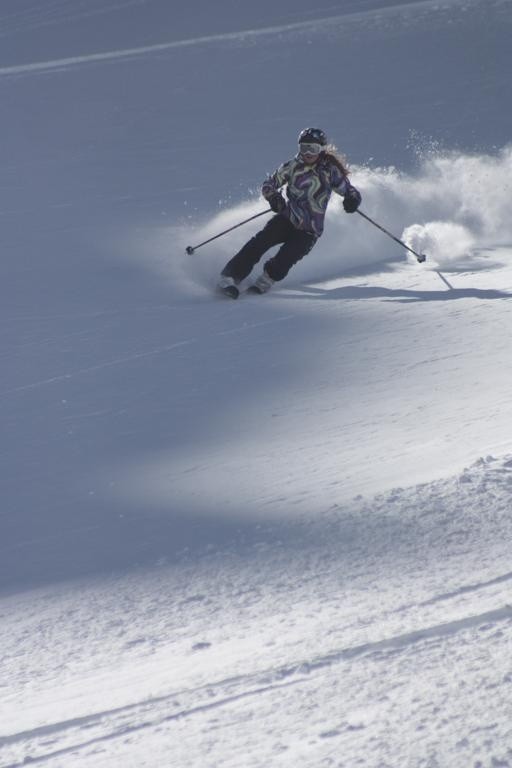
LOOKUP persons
[219,126,362,300]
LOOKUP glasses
[298,144,326,155]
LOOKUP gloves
[342,190,362,213]
[270,192,286,213]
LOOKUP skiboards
[221,285,263,300]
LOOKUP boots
[218,275,239,299]
[253,271,277,293]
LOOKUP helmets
[297,127,328,146]
